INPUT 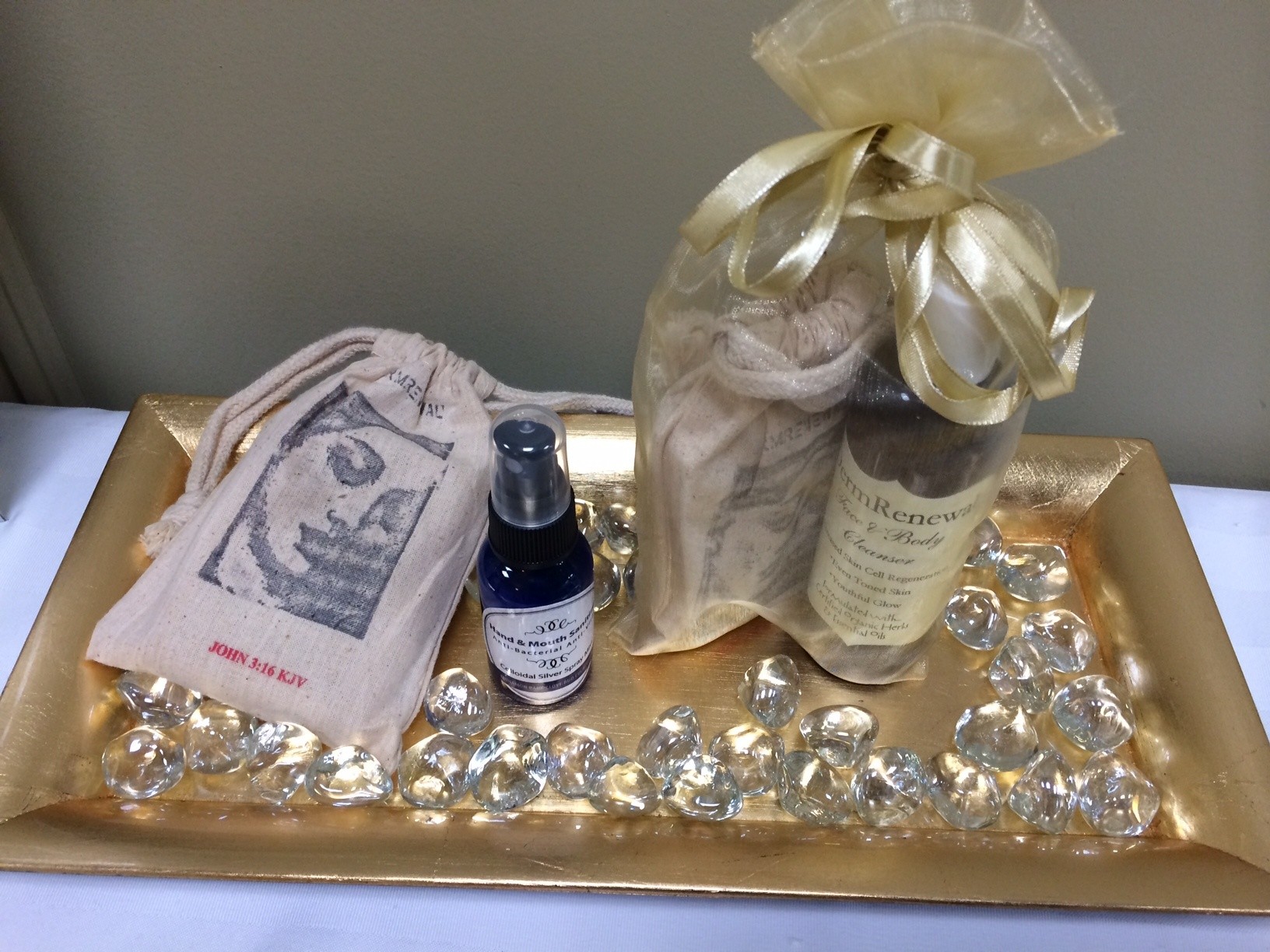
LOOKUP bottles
[476,404,600,708]
[795,273,1026,677]
